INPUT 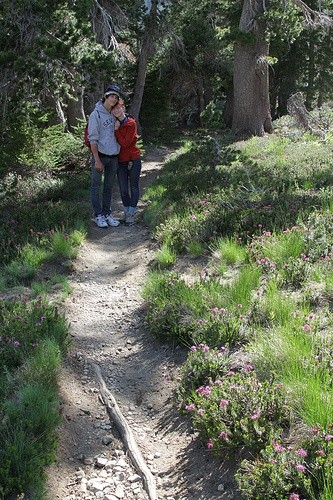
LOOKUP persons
[88,86,120,227]
[113,99,142,225]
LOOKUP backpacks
[84,110,100,148]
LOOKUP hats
[104,86,120,99]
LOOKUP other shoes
[106,214,120,226]
[120,206,138,226]
[94,214,108,228]
